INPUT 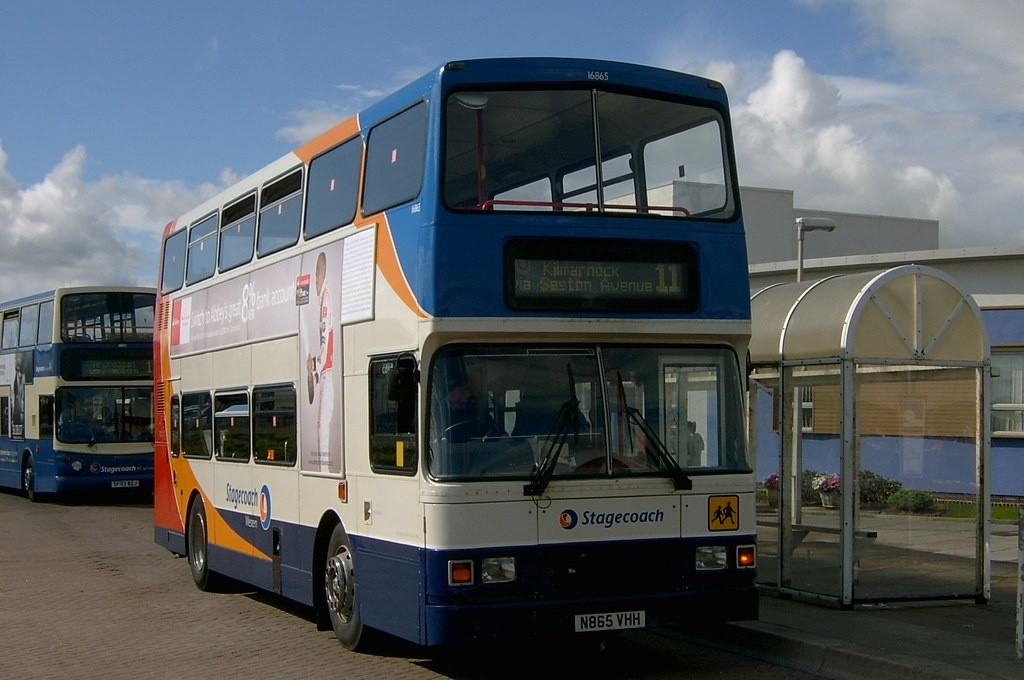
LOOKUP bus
[152,56,757,650]
[0,284,158,503]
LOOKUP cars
[149,406,249,431]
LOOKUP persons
[410,368,509,449]
[2,401,8,434]
[306,252,334,473]
[510,368,591,454]
[98,407,121,433]
[373,388,389,434]
[688,421,704,467]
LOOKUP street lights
[793,216,836,527]
